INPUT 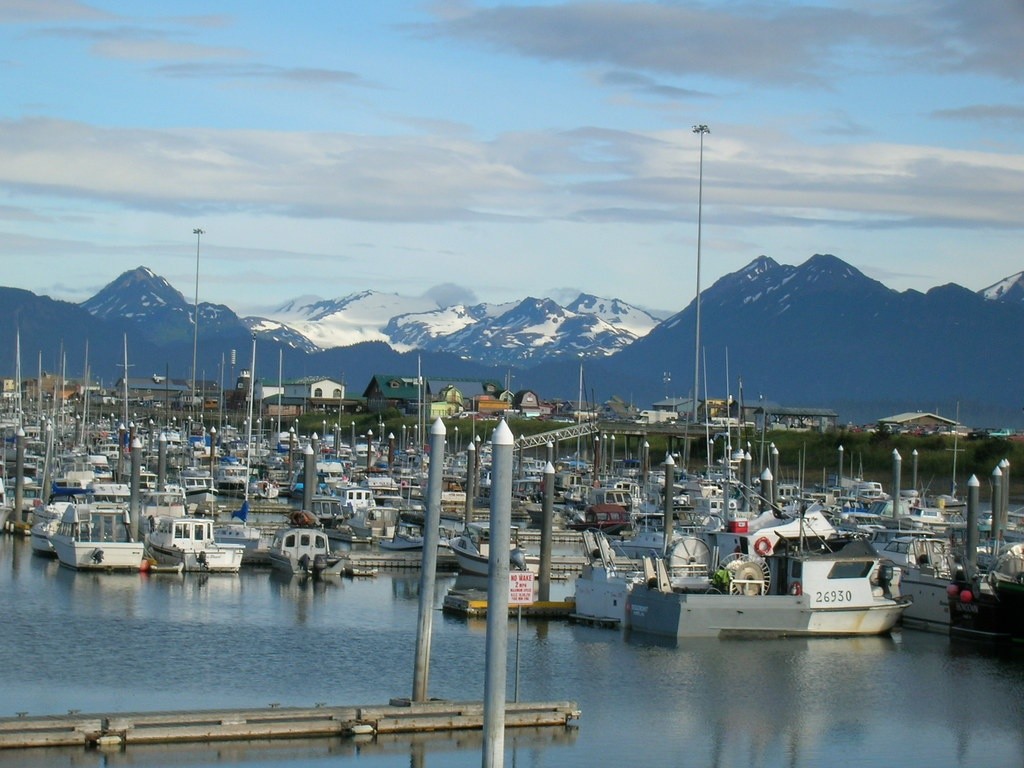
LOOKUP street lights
[188,226,203,411]
[692,123,712,424]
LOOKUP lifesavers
[754,536,772,556]
[789,581,801,595]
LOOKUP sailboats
[1,326,1024,644]
